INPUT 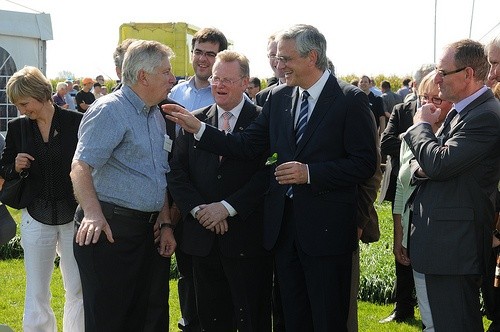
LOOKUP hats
[82,78,97,85]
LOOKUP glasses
[275,47,318,63]
[208,75,247,86]
[245,85,259,89]
[191,49,216,57]
[434,66,476,77]
[267,53,276,58]
[99,78,105,81]
[418,95,453,105]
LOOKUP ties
[222,112,234,135]
[285,91,310,201]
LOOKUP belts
[112,207,159,223]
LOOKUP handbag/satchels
[1,170,33,210]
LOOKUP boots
[376,260,415,324]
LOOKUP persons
[0,23,385,332]
[379,36,500,332]
